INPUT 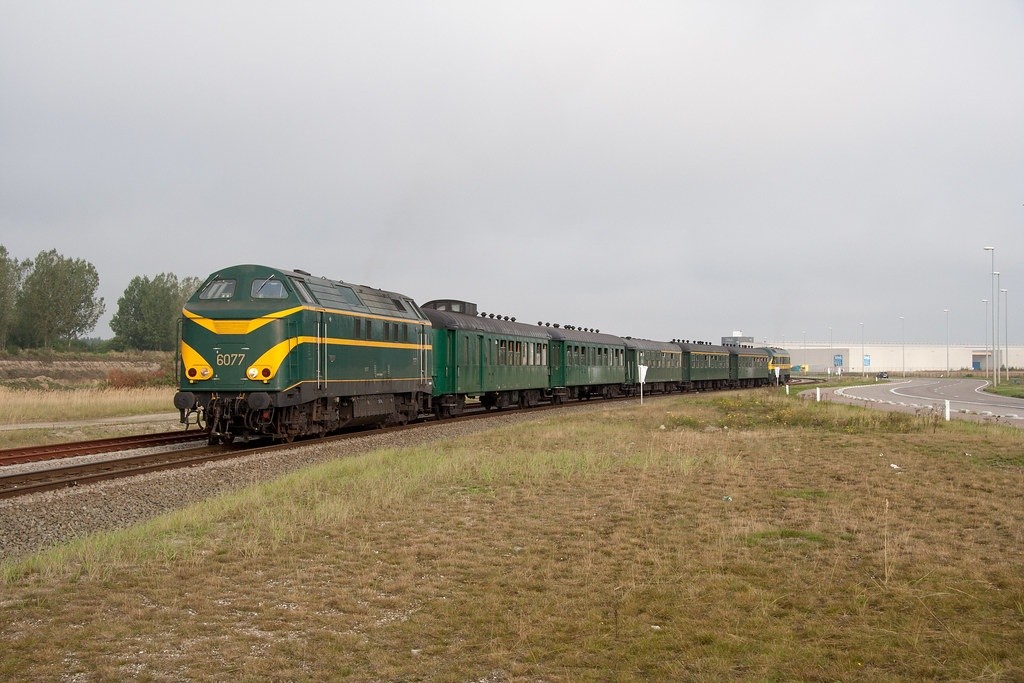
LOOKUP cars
[876,372,889,379]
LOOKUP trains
[172,263,792,441]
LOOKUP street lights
[981,299,991,378]
[944,309,950,380]
[859,323,865,374]
[763,336,768,347]
[781,334,785,350]
[992,271,1002,385]
[983,247,997,386]
[1001,289,1010,382]
[829,326,833,372]
[898,317,905,379]
[802,330,806,367]
